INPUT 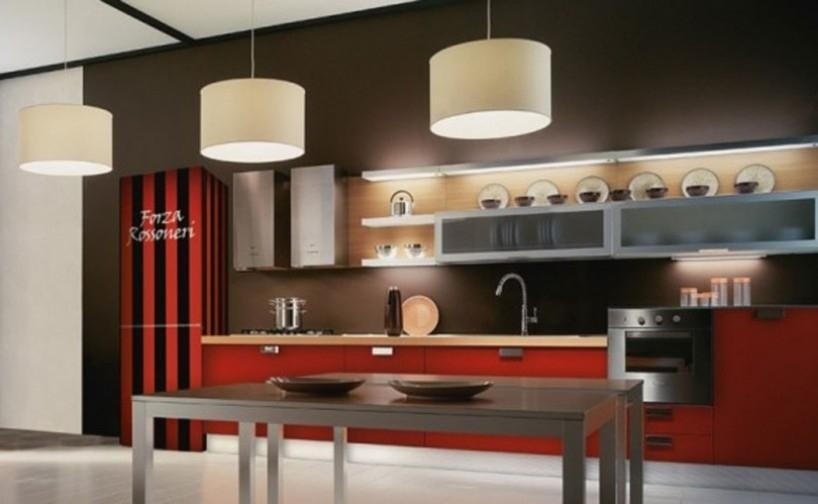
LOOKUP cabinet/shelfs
[624,406,711,492]
[428,347,608,476]
[344,344,425,464]
[362,134,817,270]
[714,308,818,493]
[203,344,339,461]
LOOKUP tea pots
[388,188,414,218]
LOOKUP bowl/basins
[578,191,600,200]
[375,243,426,260]
[515,197,534,205]
[609,189,630,199]
[549,194,566,204]
[646,188,667,198]
[687,186,709,195]
[482,198,500,209]
[735,182,758,192]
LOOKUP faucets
[493,272,540,336]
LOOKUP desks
[130,372,642,502]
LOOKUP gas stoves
[245,326,334,340]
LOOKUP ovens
[607,306,712,407]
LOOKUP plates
[269,377,364,397]
[400,296,439,336]
[525,179,559,208]
[573,176,608,202]
[628,173,665,200]
[388,378,492,404]
[477,183,508,209]
[681,168,719,197]
[734,164,776,194]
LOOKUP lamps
[199,0,306,162]
[18,1,114,178]
[428,2,551,140]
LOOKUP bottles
[385,286,402,336]
[681,277,753,307]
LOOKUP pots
[265,298,308,329]
